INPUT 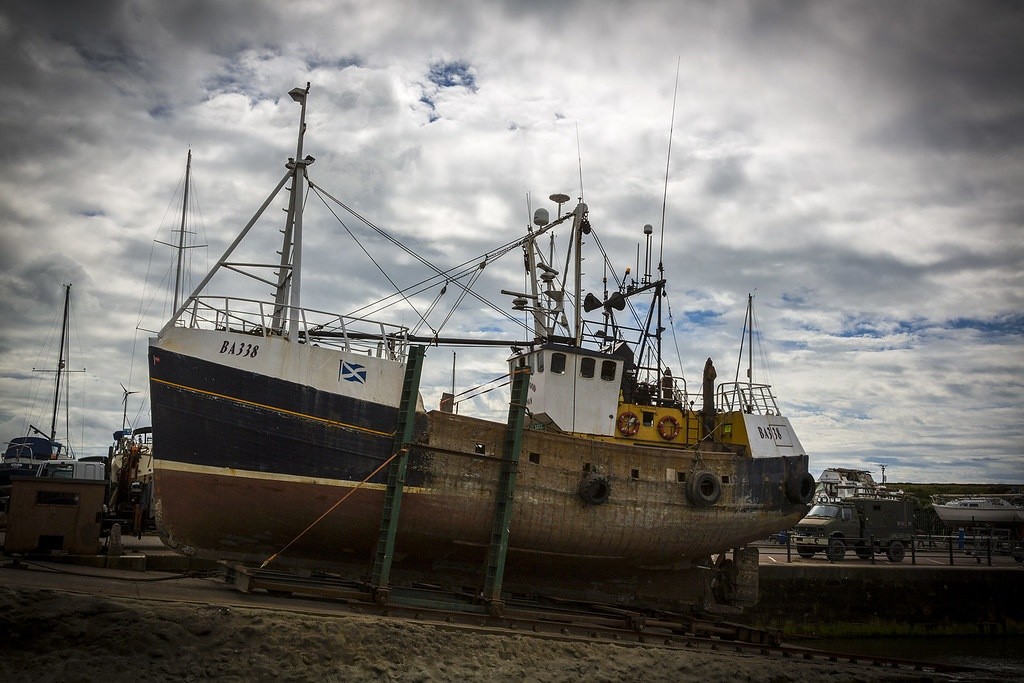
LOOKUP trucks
[794,483,916,563]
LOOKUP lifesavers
[618,412,641,436]
[686,470,722,505]
[582,473,611,504]
[657,415,681,440]
[788,472,816,504]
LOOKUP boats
[143,58,819,621]
[929,493,1024,529]
[1,279,156,542]
